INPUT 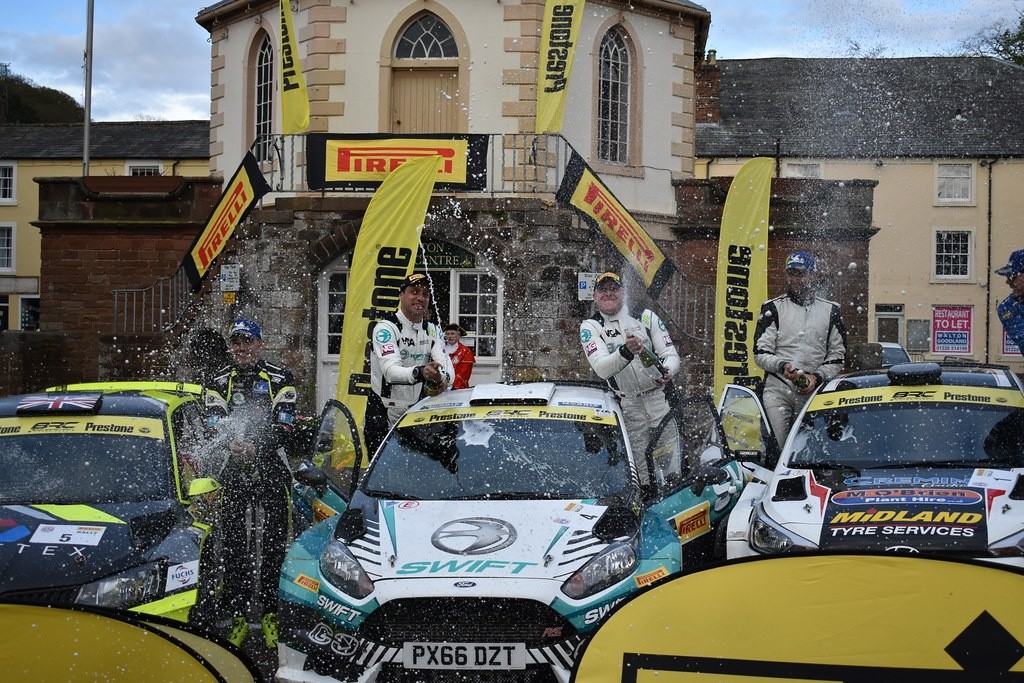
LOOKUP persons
[363,273,457,464]
[580,270,683,488]
[753,250,847,459]
[996,249,1024,359]
[442,323,476,390]
[203,317,301,650]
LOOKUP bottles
[790,370,811,393]
[629,335,668,383]
[424,370,443,396]
[237,442,266,498]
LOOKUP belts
[636,387,659,397]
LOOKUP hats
[226,320,261,340]
[993,250,1024,277]
[595,272,623,286]
[399,274,431,291]
[784,251,820,275]
[442,322,467,338]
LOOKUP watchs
[412,365,424,381]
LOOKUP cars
[274,380,747,683]
[0,388,213,612]
[713,363,1023,571]
[866,342,914,368]
